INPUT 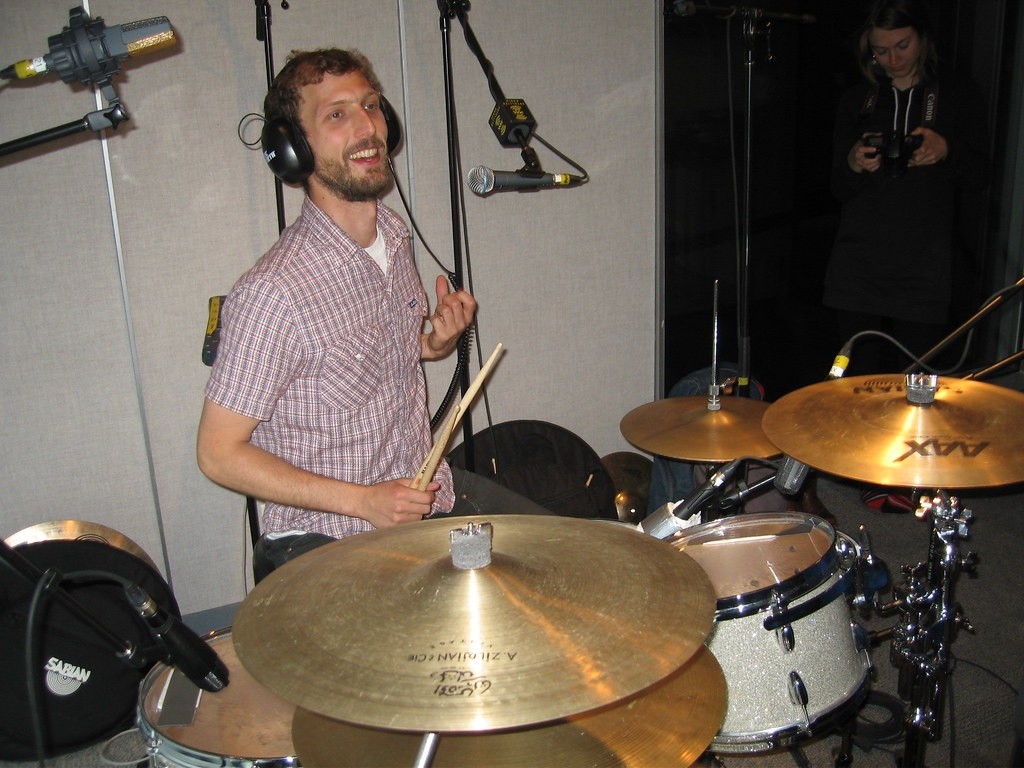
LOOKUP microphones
[125,582,229,693]
[639,458,745,541]
[0,16,177,80]
[468,165,582,194]
[774,341,853,495]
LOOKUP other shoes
[783,478,837,528]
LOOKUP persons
[196,54,558,589]
[813,0,989,513]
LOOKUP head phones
[258,57,400,184]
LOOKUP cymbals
[290,644,730,768]
[618,396,783,463]
[232,513,718,735]
[3,519,162,580]
[760,373,1024,489]
[600,451,655,523]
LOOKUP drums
[135,626,300,768]
[660,509,877,754]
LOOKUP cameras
[866,133,924,176]
